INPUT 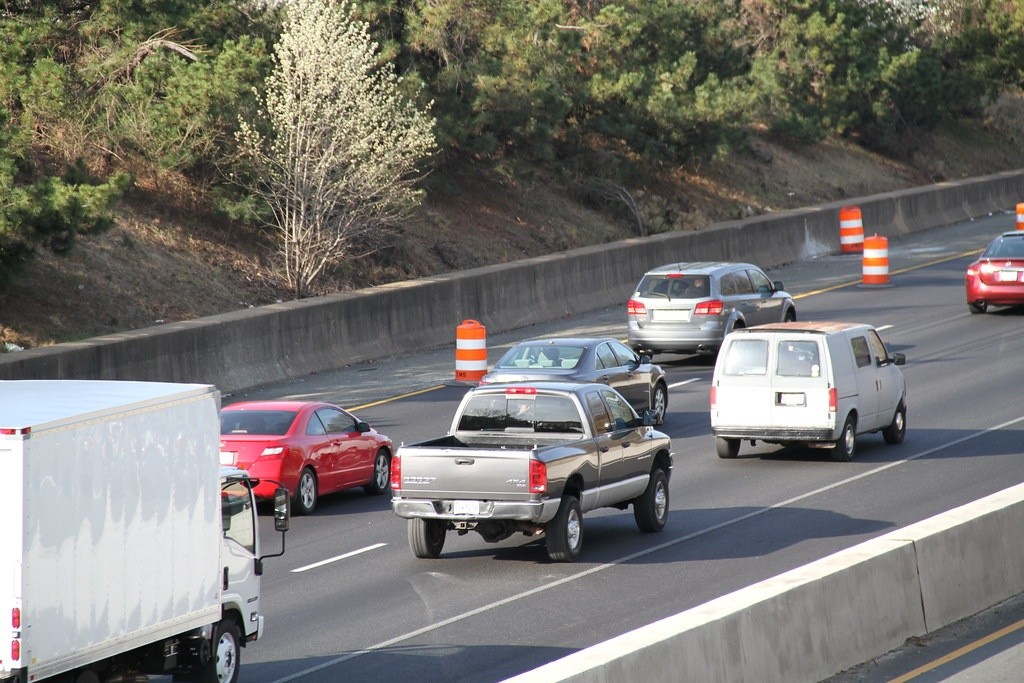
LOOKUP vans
[709,321,908,462]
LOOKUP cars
[220,399,394,517]
[965,230,1024,316]
[477,337,669,430]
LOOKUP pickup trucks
[390,381,674,563]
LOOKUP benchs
[512,359,579,368]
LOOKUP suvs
[627,260,796,365]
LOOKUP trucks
[0,379,290,683]
